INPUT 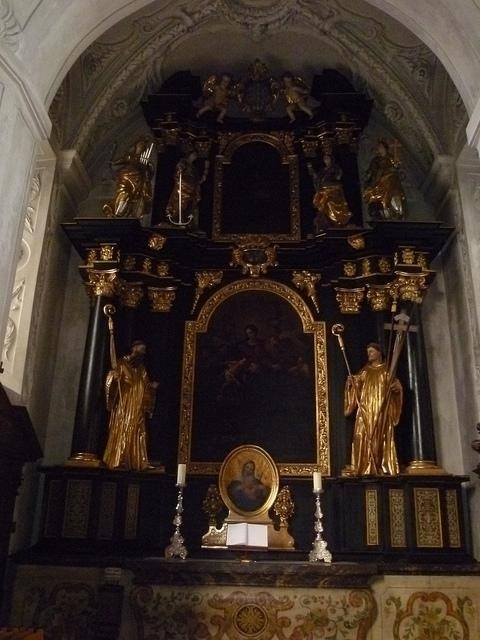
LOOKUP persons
[197,74,236,123]
[360,139,405,219]
[340,339,403,478]
[311,153,351,229]
[103,138,153,218]
[102,342,160,470]
[231,323,266,360]
[169,146,199,225]
[273,72,313,123]
[226,461,269,511]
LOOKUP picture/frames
[176,278,333,479]
[218,444,282,515]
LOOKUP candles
[311,462,324,492]
[178,462,187,488]
